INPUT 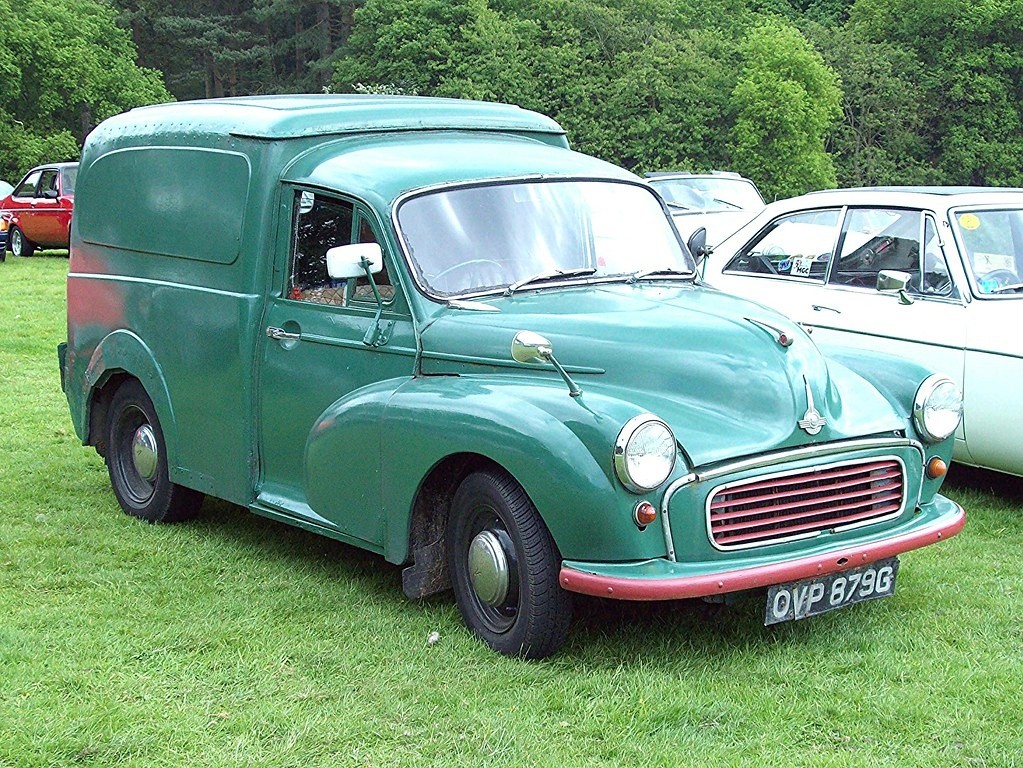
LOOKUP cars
[57,96,967,659]
[690,185,1023,487]
[641,170,768,263]
[0,162,78,257]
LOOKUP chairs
[50,174,73,195]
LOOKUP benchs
[792,265,920,286]
